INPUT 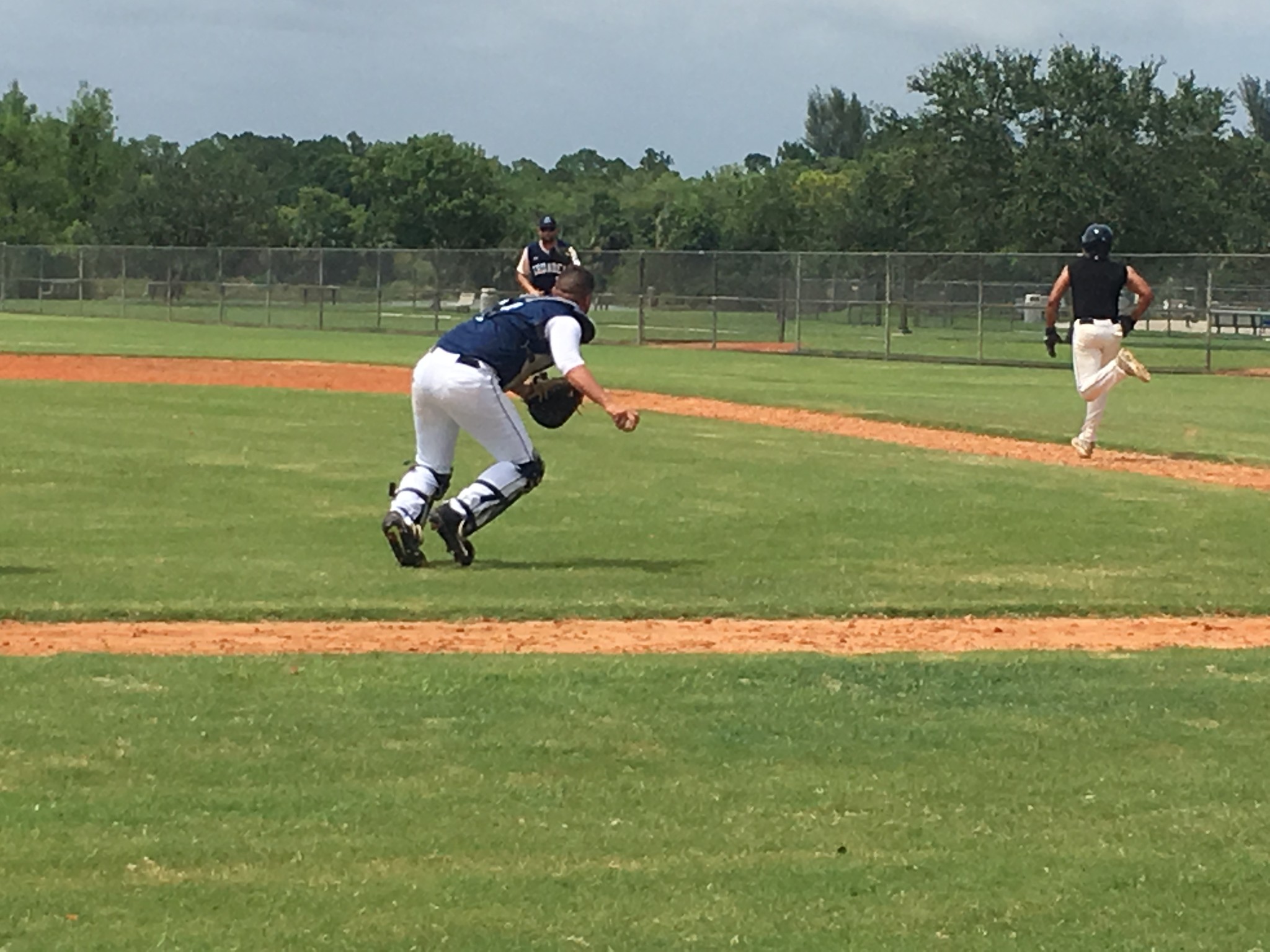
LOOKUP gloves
[1116,313,1135,339]
[1043,325,1062,360]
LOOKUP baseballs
[623,420,636,431]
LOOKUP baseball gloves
[528,378,585,430]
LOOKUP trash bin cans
[1025,294,1042,322]
[480,287,496,313]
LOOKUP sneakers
[382,512,427,567]
[1071,437,1090,459]
[429,503,475,566]
[1117,347,1152,383]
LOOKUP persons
[1044,224,1155,458]
[516,216,582,295]
[380,265,639,567]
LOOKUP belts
[1079,316,1094,324]
[457,355,480,369]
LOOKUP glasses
[540,227,555,232]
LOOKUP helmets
[1082,223,1114,253]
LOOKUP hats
[540,215,556,229]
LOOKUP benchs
[454,292,475,313]
